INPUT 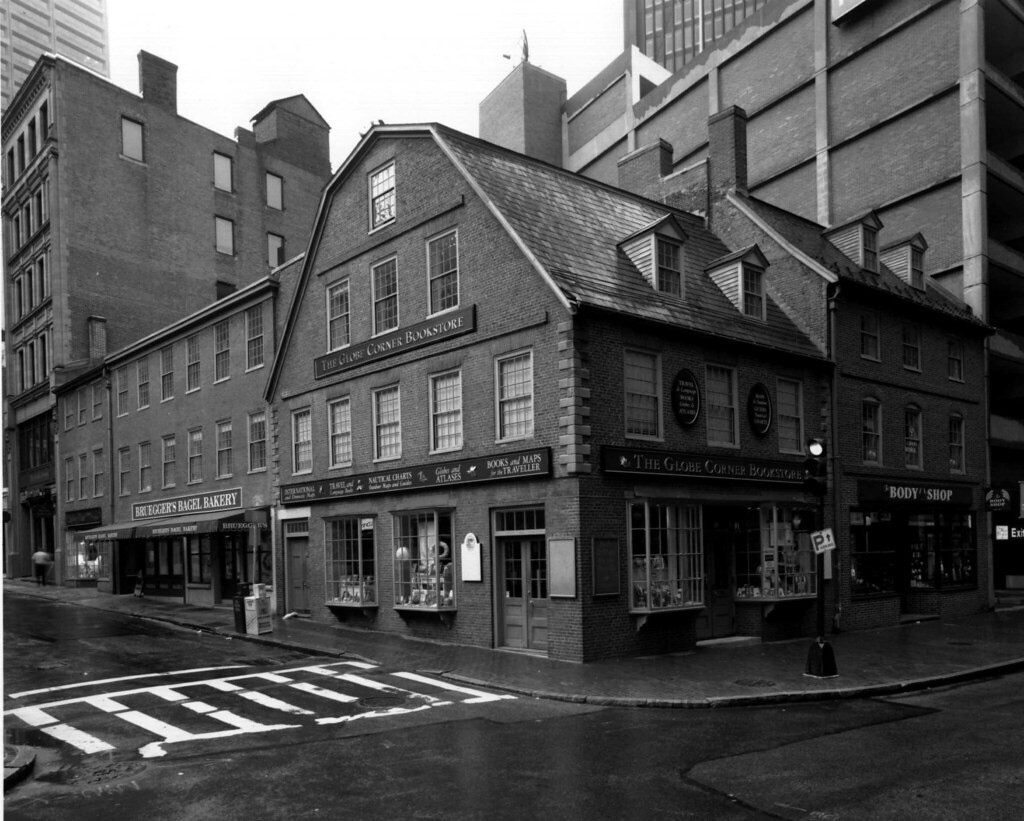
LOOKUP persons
[32,548,50,585]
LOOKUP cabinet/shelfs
[909,541,927,583]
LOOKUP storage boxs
[244,584,273,636]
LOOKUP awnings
[73,506,263,543]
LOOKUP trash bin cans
[231,581,273,636]
